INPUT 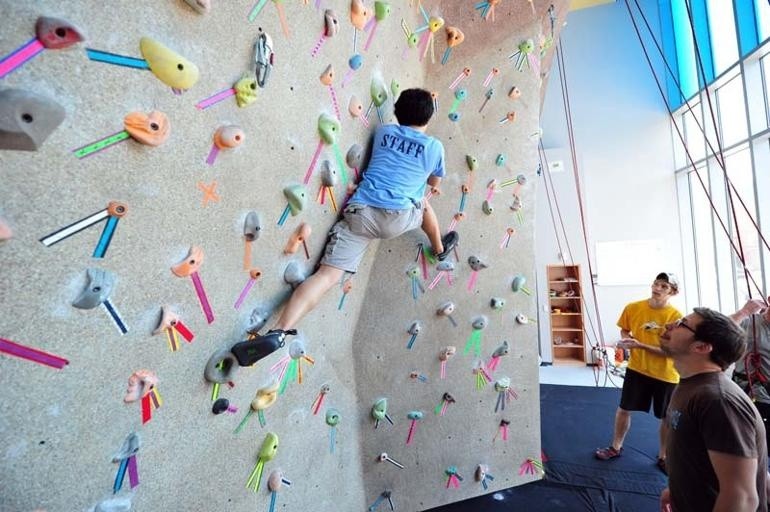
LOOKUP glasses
[675,319,695,335]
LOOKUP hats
[656,272,679,284]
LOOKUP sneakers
[433,231,459,261]
[592,446,624,460]
[656,458,666,475]
[231,329,286,366]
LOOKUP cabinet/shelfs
[546,263,587,364]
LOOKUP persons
[659,306,770,512]
[233,88,459,367]
[728,296,770,460]
[595,272,684,473]
